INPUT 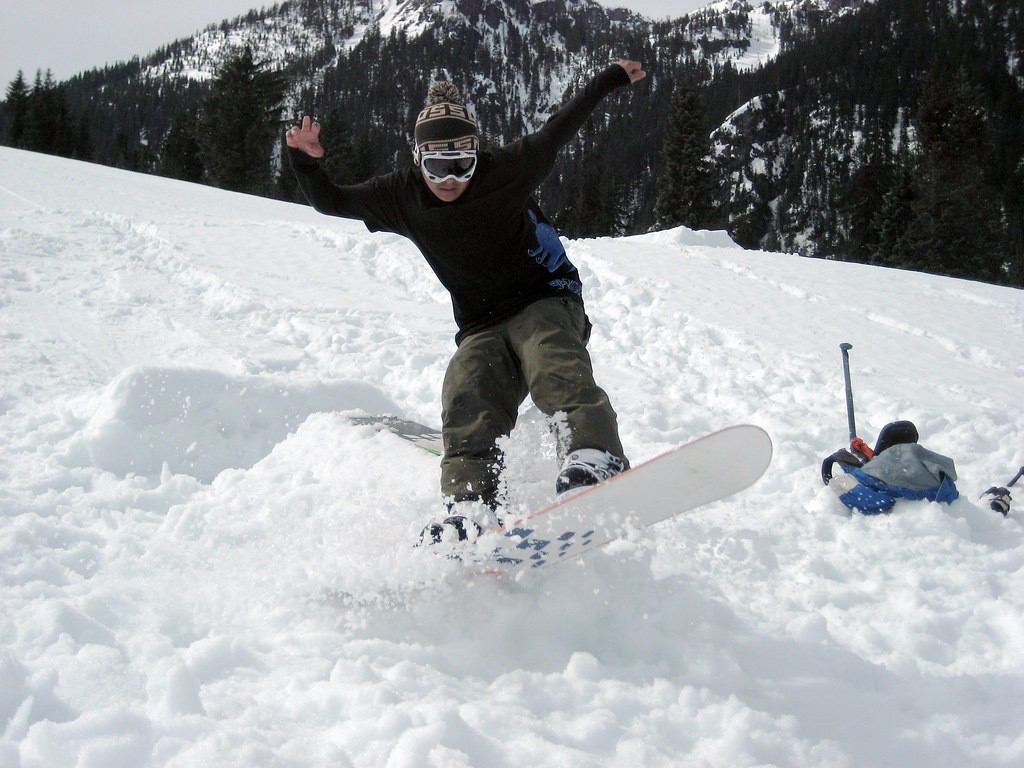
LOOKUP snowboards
[436,425,773,575]
[352,415,445,456]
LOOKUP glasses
[419,151,477,183]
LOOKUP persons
[284,59,648,543]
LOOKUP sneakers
[419,515,503,544]
[554,463,612,495]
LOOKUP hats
[414,82,480,151]
